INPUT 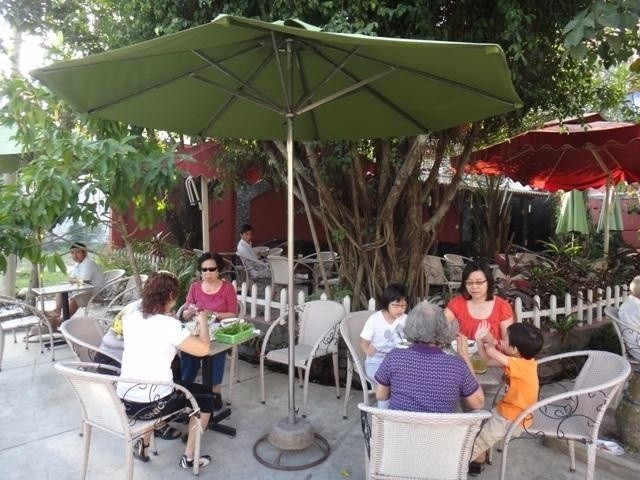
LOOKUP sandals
[153,423,182,441]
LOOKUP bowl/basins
[394,339,412,351]
[220,318,245,328]
[207,314,216,325]
[451,339,479,357]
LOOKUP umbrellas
[31,14,525,425]
[556,189,589,259]
[449,112,640,257]
[597,186,625,233]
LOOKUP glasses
[200,267,218,273]
[389,302,410,309]
[465,280,487,287]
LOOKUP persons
[470,319,544,463]
[38,243,105,328]
[373,297,485,413]
[116,269,211,468]
[618,274,640,359]
[95,305,181,439]
[237,224,271,278]
[440,258,514,350]
[184,250,238,394]
[360,282,408,408]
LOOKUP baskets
[215,323,255,344]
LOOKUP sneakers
[133,437,150,463]
[212,391,223,412]
[178,454,212,469]
